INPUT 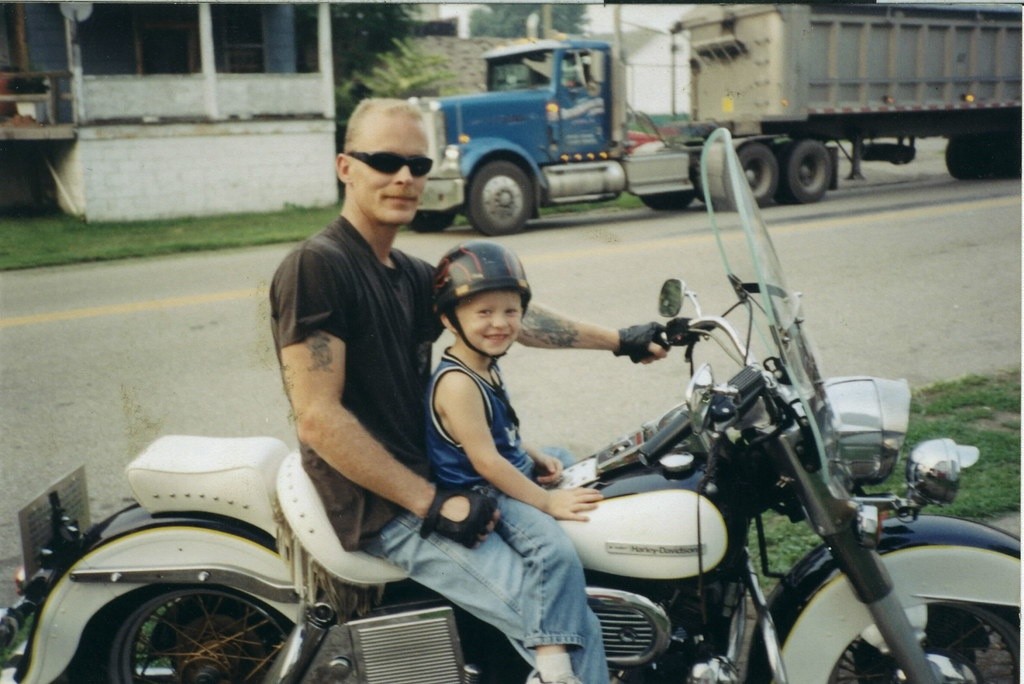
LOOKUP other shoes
[528,672,584,684]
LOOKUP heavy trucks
[407,5,1022,236]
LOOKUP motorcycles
[0,126,1022,683]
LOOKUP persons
[269,98,669,684]
[426,240,603,684]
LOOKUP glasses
[343,151,432,176]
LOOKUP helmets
[433,241,532,323]
